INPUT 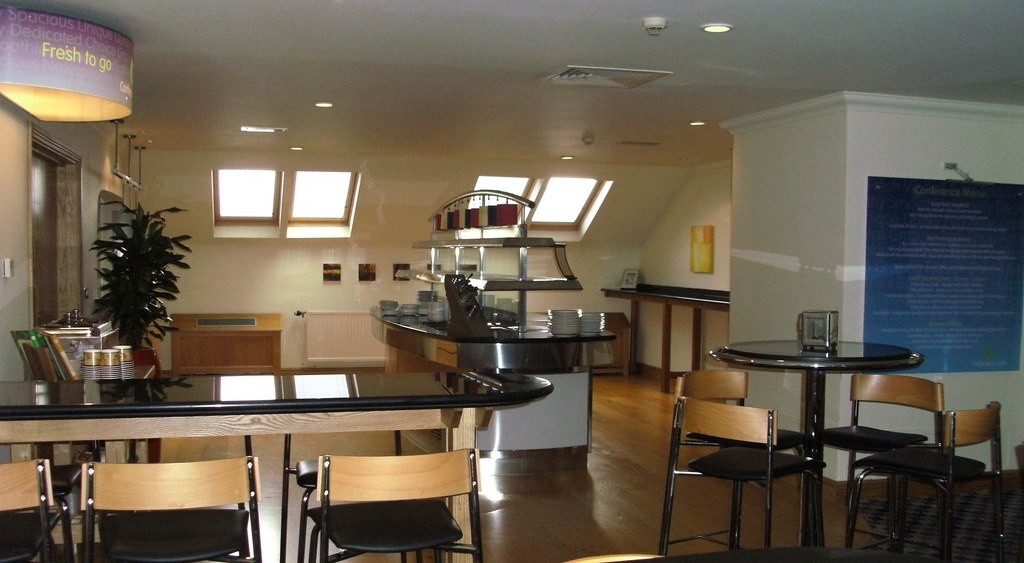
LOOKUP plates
[546,308,605,336]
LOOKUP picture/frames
[621,270,638,289]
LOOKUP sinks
[502,319,549,334]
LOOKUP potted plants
[86,200,192,462]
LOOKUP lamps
[107,118,145,193]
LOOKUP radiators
[295,310,387,362]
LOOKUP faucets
[492,312,501,326]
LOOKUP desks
[0,373,554,563]
[704,340,924,549]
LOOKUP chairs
[823,372,945,549]
[846,403,1004,563]
[280,432,483,563]
[657,393,828,557]
[0,434,263,563]
[674,370,806,549]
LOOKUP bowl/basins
[80,345,134,380]
